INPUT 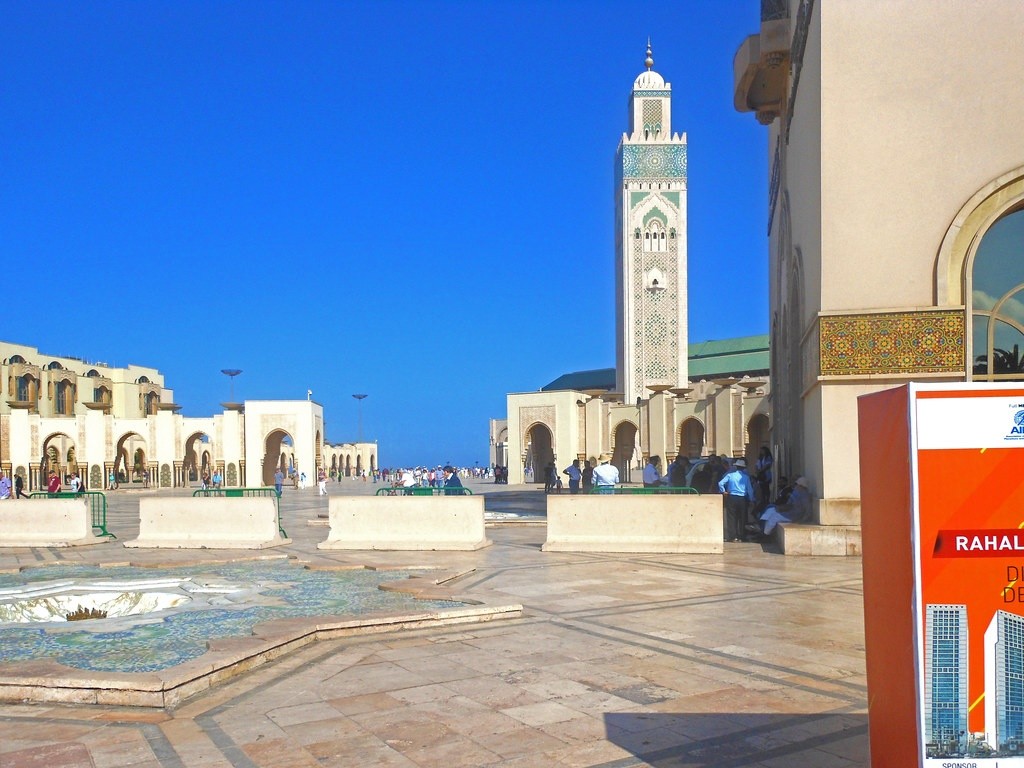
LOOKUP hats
[733,460,747,467]
[598,454,611,463]
[795,476,808,488]
[48,470,54,474]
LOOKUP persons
[373,465,494,496]
[330,466,367,484]
[643,446,811,543]
[545,453,620,495]
[48,470,85,493]
[137,470,149,488]
[203,471,222,497]
[494,464,508,484]
[273,468,327,497]
[107,473,115,490]
[0,471,28,499]
[524,466,533,477]
